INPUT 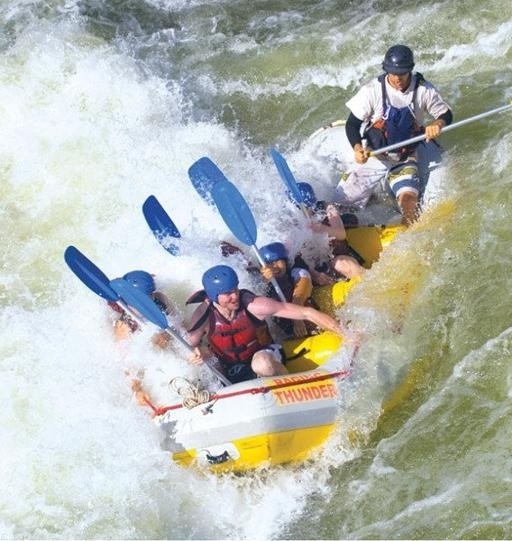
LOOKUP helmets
[125,269,157,295]
[202,266,241,302]
[257,242,288,263]
[381,44,415,74]
[288,182,318,209]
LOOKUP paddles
[212,180,289,303]
[188,156,229,206]
[269,147,313,226]
[142,194,183,258]
[108,278,234,385]
[65,245,145,326]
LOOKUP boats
[150,121,454,478]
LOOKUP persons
[334,45,453,228]
[184,266,341,387]
[285,183,371,285]
[258,243,321,339]
[114,271,223,416]
